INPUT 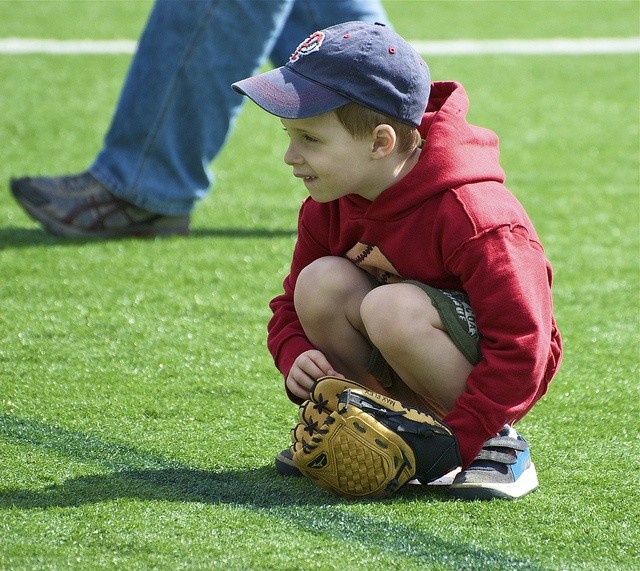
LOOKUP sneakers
[275,448,303,477]
[11,171,189,240]
[451,423,539,500]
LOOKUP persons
[9,3,397,242]
[230,21,564,501]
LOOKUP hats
[231,20,431,127]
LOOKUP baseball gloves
[289,375,463,500]
